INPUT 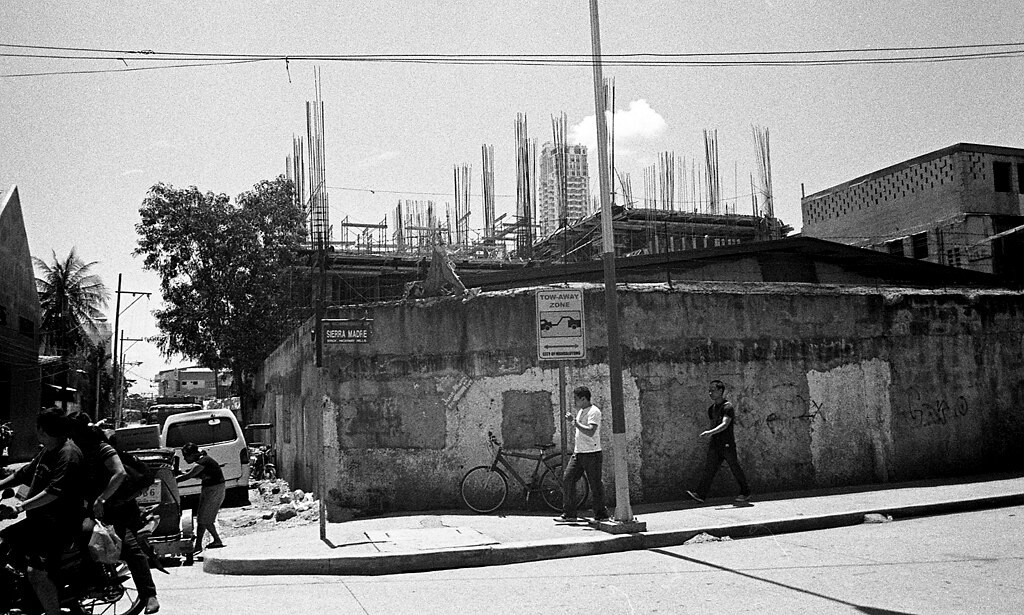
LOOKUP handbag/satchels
[102,448,156,512]
[88,516,126,566]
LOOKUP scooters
[248,444,277,482]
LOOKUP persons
[686,380,752,502]
[174,442,226,555]
[0,404,159,615]
[553,385,608,523]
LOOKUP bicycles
[460,434,590,514]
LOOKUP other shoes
[553,513,579,523]
[204,542,224,548]
[686,490,706,504]
[588,518,609,526]
[734,494,754,503]
[144,596,162,614]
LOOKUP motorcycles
[0,442,196,615]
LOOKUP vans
[160,407,254,510]
[140,402,204,435]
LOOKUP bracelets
[571,419,576,426]
